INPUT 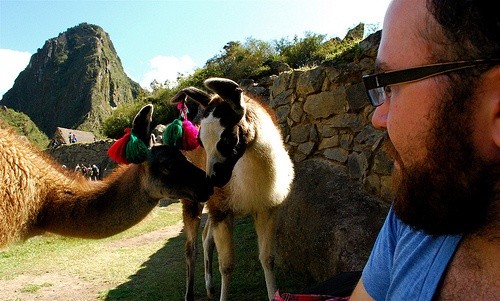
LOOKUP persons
[345,1,500,301]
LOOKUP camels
[170,75,297,301]
[0,104,214,253]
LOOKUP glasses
[362,58,500,108]
